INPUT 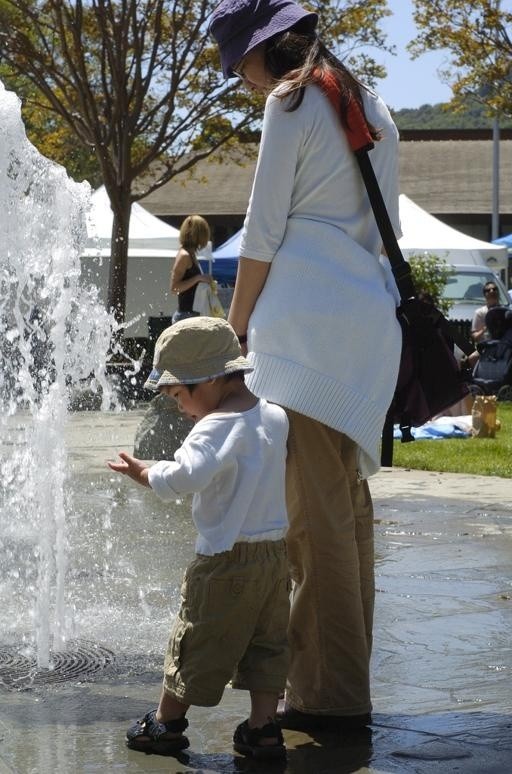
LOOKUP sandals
[125,709,190,752]
[233,716,287,761]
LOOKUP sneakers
[143,377,159,396]
[275,700,375,727]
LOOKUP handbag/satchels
[390,297,472,427]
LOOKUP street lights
[479,52,511,240]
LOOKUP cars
[412,265,511,321]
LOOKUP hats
[209,1,318,80]
[142,318,252,392]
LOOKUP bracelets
[235,333,249,345]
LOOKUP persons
[209,0,407,768]
[132,213,223,459]
[108,316,293,762]
[467,281,501,370]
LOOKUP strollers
[463,307,511,400]
